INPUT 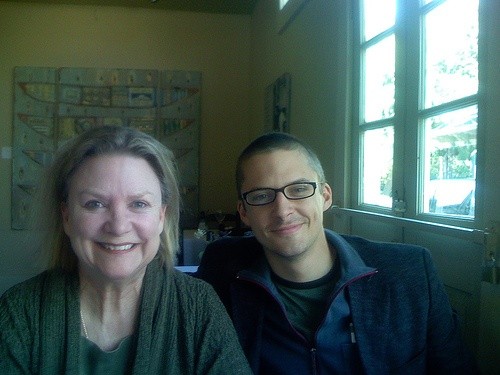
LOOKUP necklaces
[79,310,90,339]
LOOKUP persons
[193,132,463,375]
[0,125,254,375]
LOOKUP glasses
[242,182,322,207]
